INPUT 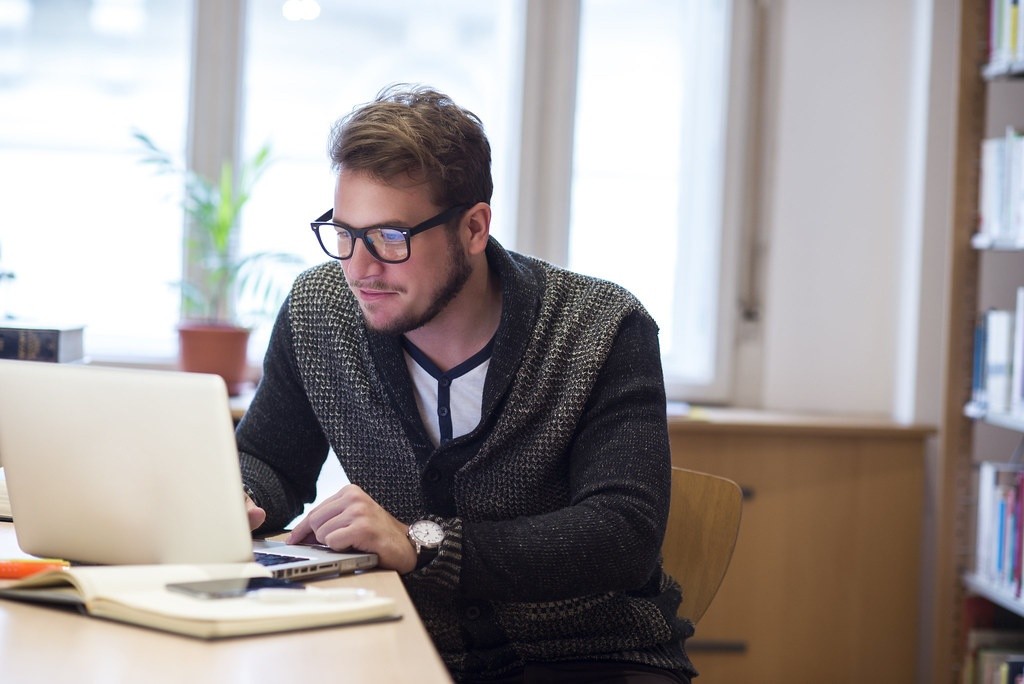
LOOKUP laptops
[0,359,379,591]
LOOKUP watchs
[406,518,446,573]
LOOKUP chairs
[656,465,743,624]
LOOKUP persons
[234,82,704,684]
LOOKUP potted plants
[132,130,307,396]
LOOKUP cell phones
[165,576,306,598]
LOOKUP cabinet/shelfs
[940,0,1024,684]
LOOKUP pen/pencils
[10,559,71,567]
[0,562,68,578]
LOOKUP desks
[0,512,454,684]
[666,404,940,684]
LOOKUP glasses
[310,203,469,264]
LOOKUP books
[964,0,1024,684]
[0,320,89,523]
[0,561,404,638]
